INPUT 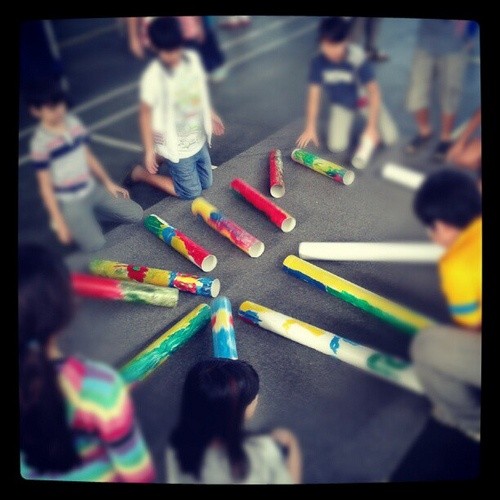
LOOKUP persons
[19,239,155,484]
[444,107,481,170]
[125,17,230,84]
[29,85,144,251]
[166,356,302,484]
[296,17,397,153]
[122,17,223,200]
[409,168,484,447]
[406,20,469,161]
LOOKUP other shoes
[121,164,140,188]
[365,43,389,62]
[432,137,456,159]
[405,131,434,154]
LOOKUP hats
[27,73,75,108]
